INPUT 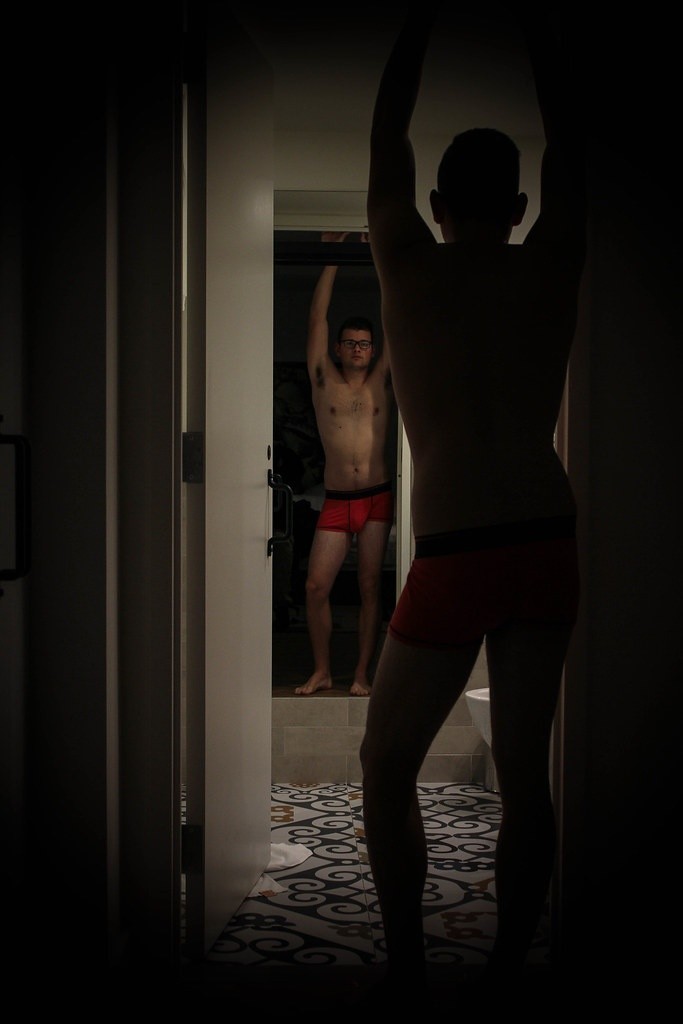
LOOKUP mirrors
[272,223,405,697]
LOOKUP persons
[326,1,586,1024]
[294,231,394,695]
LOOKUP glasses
[340,339,373,350]
